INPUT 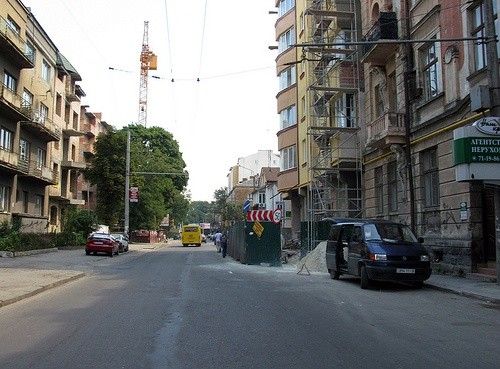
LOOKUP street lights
[122,125,138,237]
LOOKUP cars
[208,233,227,250]
[201,234,207,243]
[85,233,120,257]
[110,234,130,253]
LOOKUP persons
[220,234,228,258]
[215,231,222,253]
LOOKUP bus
[182,225,202,247]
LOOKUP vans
[323,216,432,289]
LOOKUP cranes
[138,20,159,129]
